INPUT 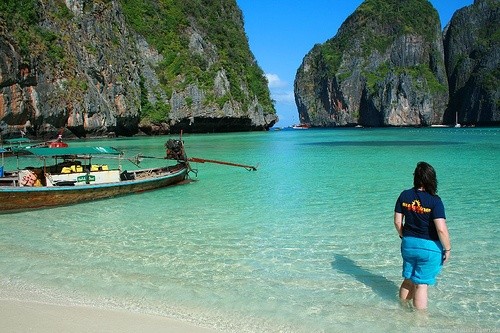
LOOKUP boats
[0,129,198,211]
[0,132,125,159]
[292,123,309,129]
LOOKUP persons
[394,162,451,311]
[57,135,62,143]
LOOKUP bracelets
[399,235,403,239]
[442,247,451,251]
